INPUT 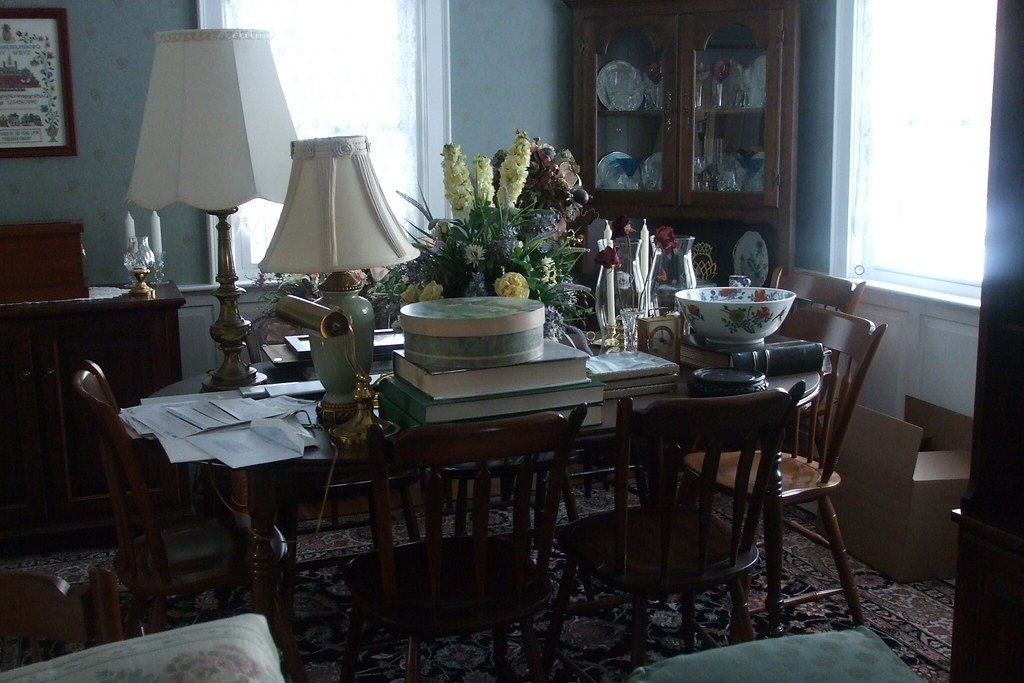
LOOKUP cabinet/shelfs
[565,0,803,286]
[0,281,200,559]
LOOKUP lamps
[127,29,299,392]
[258,132,421,429]
[275,294,400,451]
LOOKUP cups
[729,275,751,286]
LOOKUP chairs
[72,264,888,683]
[1,566,124,665]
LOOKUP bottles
[646,235,696,336]
[596,238,645,356]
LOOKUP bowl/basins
[675,286,797,344]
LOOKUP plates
[598,152,641,191]
[597,60,645,111]
[748,55,767,108]
[744,152,764,192]
[642,152,663,190]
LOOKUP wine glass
[643,162,658,190]
[606,62,664,111]
[696,60,767,108]
[598,159,640,190]
[695,157,706,192]
[736,158,764,192]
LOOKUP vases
[620,306,650,354]
[648,236,697,318]
[594,239,644,355]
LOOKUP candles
[640,219,649,311]
[150,210,163,252]
[125,211,135,251]
[604,221,616,326]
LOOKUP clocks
[638,307,682,363]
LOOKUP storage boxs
[1,218,89,303]
[816,396,973,583]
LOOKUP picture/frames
[0,7,78,157]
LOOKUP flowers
[243,127,680,358]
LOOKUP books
[679,333,824,377]
[377,339,607,434]
